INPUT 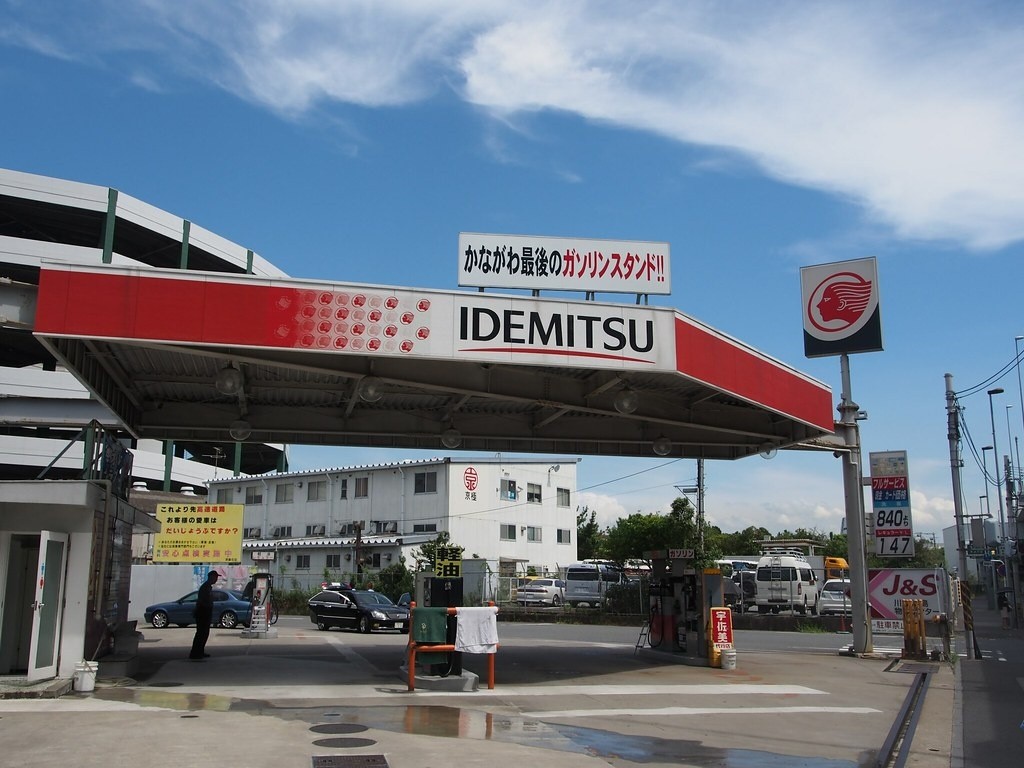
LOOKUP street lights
[981,446,994,513]
[1015,335,1024,427]
[988,387,1006,540]
[1014,435,1021,481]
[1005,404,1015,494]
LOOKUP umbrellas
[996,586,1015,595]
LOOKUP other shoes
[189,653,209,660]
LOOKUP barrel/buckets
[677,627,687,647]
[73,661,98,691]
[721,649,737,669]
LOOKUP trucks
[721,555,849,600]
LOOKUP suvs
[818,578,852,616]
[307,589,412,635]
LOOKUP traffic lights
[990,549,997,556]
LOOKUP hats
[208,570,222,577]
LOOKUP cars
[144,589,274,629]
[518,579,566,607]
[509,576,544,601]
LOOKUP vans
[754,557,818,617]
[565,563,629,609]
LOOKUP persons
[189,570,222,659]
[997,592,1011,630]
